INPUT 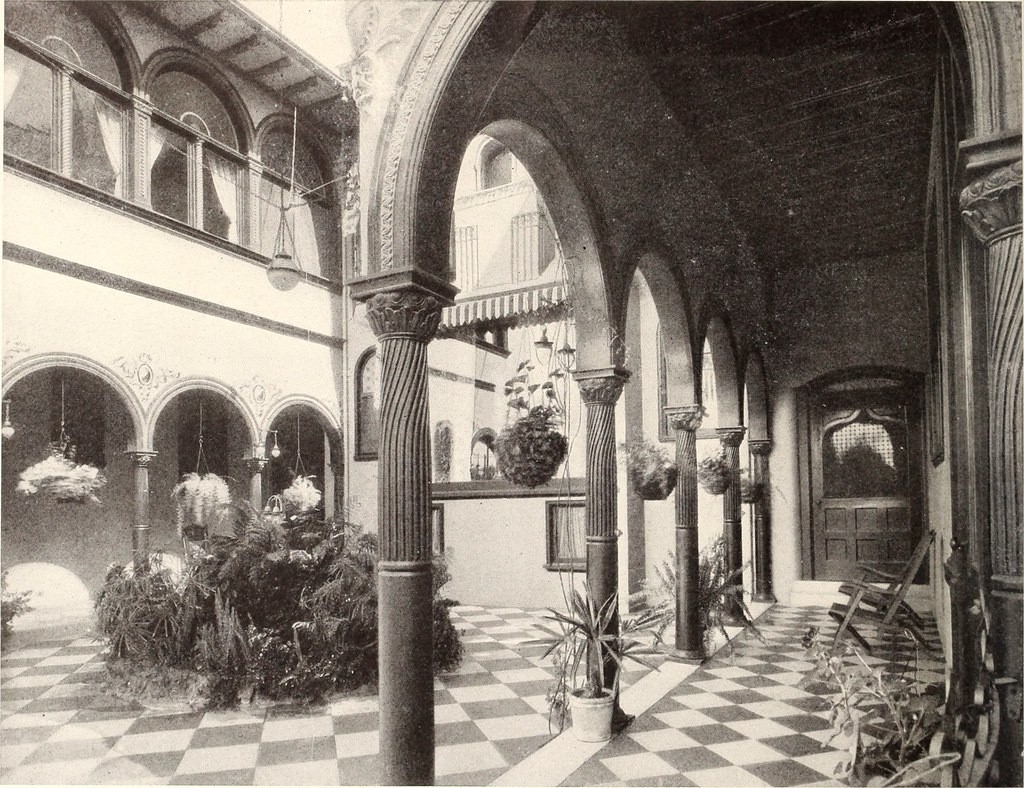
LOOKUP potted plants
[530,579,664,744]
[17,457,107,508]
[699,446,734,496]
[493,360,570,489]
[629,541,769,654]
[171,472,237,541]
[615,426,680,502]
[282,475,323,511]
[740,465,772,503]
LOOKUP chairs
[828,529,937,654]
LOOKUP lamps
[1,399,14,440]
[268,429,280,457]
